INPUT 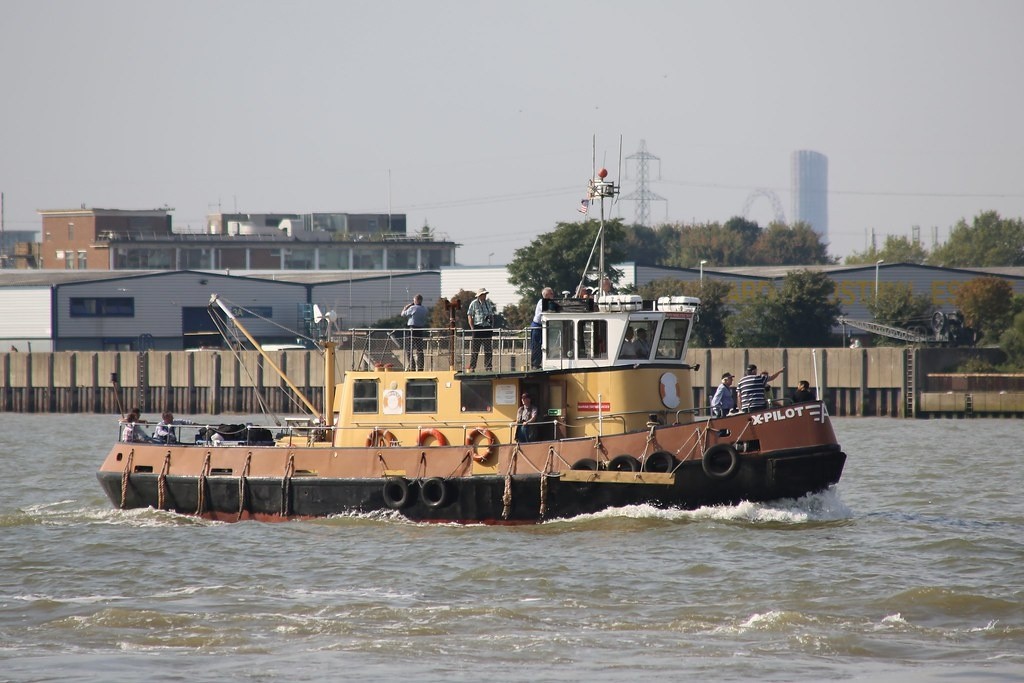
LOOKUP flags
[576,199,588,213]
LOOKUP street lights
[875,258,885,320]
[700,258,708,285]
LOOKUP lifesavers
[607,456,638,488]
[421,477,447,508]
[383,477,409,509]
[417,430,446,447]
[367,430,395,447]
[467,429,495,463]
[571,459,599,492]
[644,450,674,473]
[702,444,737,479]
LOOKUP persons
[634,328,649,357]
[849,337,862,349]
[620,326,636,355]
[736,364,786,412]
[595,277,619,296]
[400,294,428,371]
[153,410,198,442]
[119,408,149,442]
[790,381,815,404]
[760,370,782,407]
[467,287,494,372]
[571,285,593,350]
[509,392,538,443]
[530,287,554,370]
[711,372,735,415]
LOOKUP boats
[95,133,846,527]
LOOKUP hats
[520,393,531,399]
[747,364,756,372]
[475,288,489,297]
[723,373,735,379]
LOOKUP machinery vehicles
[833,309,980,346]
